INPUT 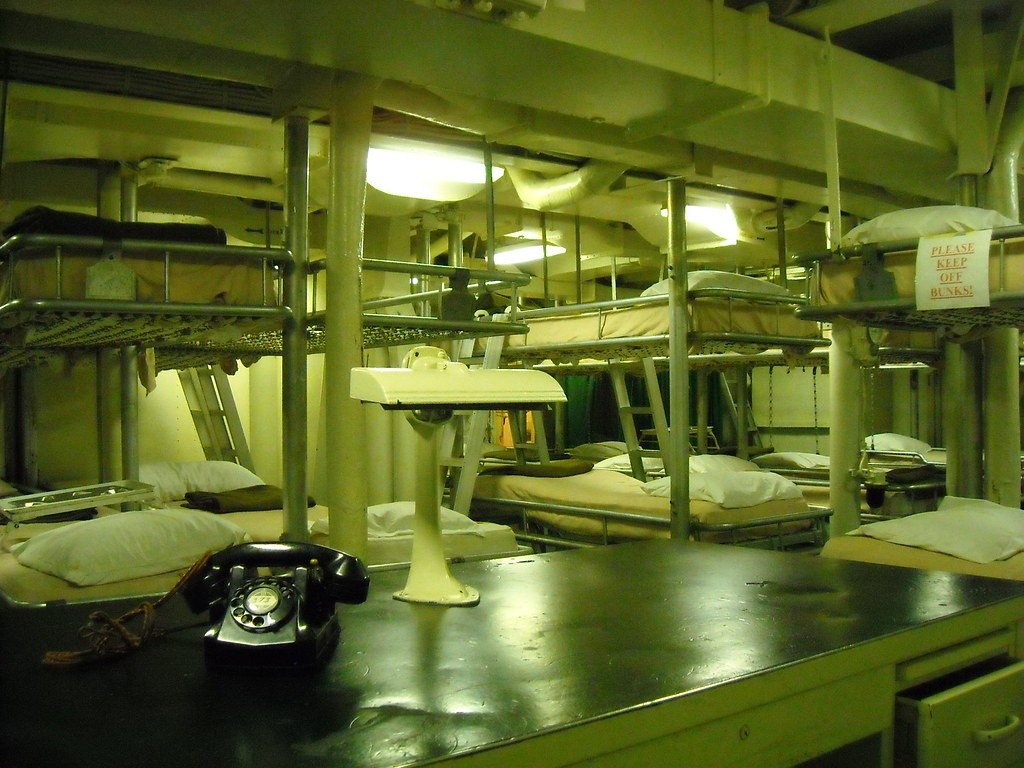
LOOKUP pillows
[9,508,246,587]
[845,496,1024,562]
[570,440,642,459]
[658,454,762,473]
[751,452,830,470]
[306,500,487,539]
[864,432,931,455]
[843,203,1021,245]
[634,471,803,508]
[640,270,791,304]
[594,450,664,472]
[136,460,266,504]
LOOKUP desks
[0,537,1024,767]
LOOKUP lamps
[483,240,567,267]
[349,344,570,607]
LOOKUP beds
[1,228,1024,610]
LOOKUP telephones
[183,541,371,685]
